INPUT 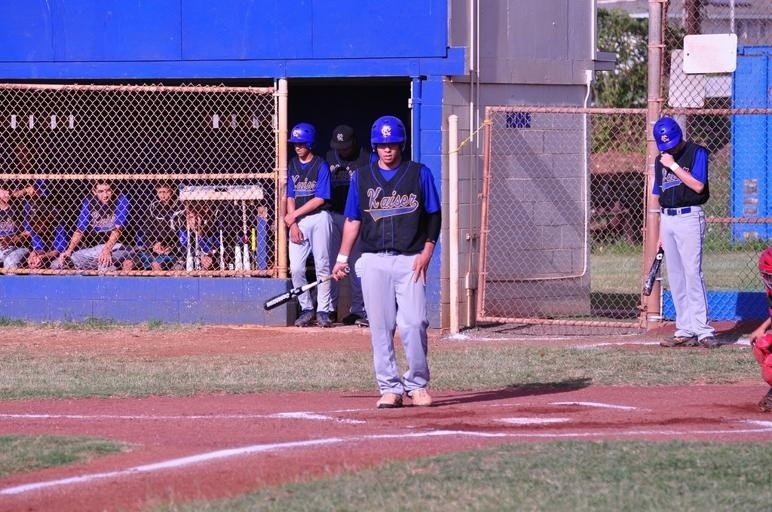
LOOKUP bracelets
[670,162,679,171]
[336,252,347,265]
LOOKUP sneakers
[697,335,724,349]
[660,336,699,346]
[295,310,433,406]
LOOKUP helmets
[285,115,408,154]
[756,247,771,296]
[653,117,683,152]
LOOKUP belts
[661,207,691,216]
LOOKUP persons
[1,142,276,277]
[284,123,334,327]
[331,114,442,408]
[750,243,771,412]
[321,124,371,326]
[651,115,722,347]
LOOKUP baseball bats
[642,248,663,297]
[183,198,261,276]
[263,266,350,310]
